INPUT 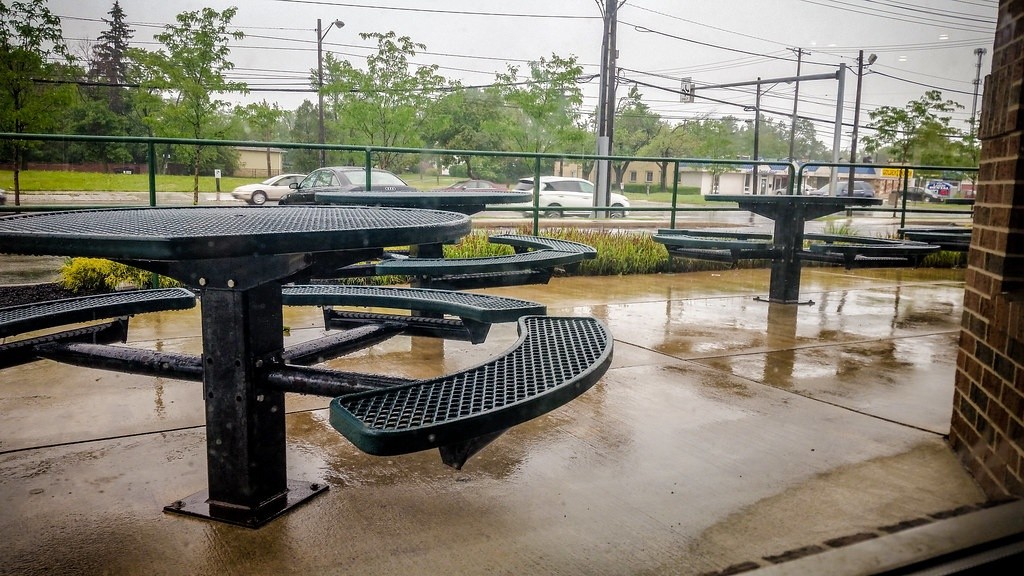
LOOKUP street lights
[316,17,345,168]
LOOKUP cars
[230,174,329,205]
[511,175,632,219]
[773,182,817,195]
[889,186,945,203]
[429,179,509,193]
[278,165,409,206]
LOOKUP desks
[314,188,533,317]
[0,206,472,530]
[705,194,883,302]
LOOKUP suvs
[819,181,876,198]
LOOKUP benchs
[650,227,974,268]
[0,234,615,471]
